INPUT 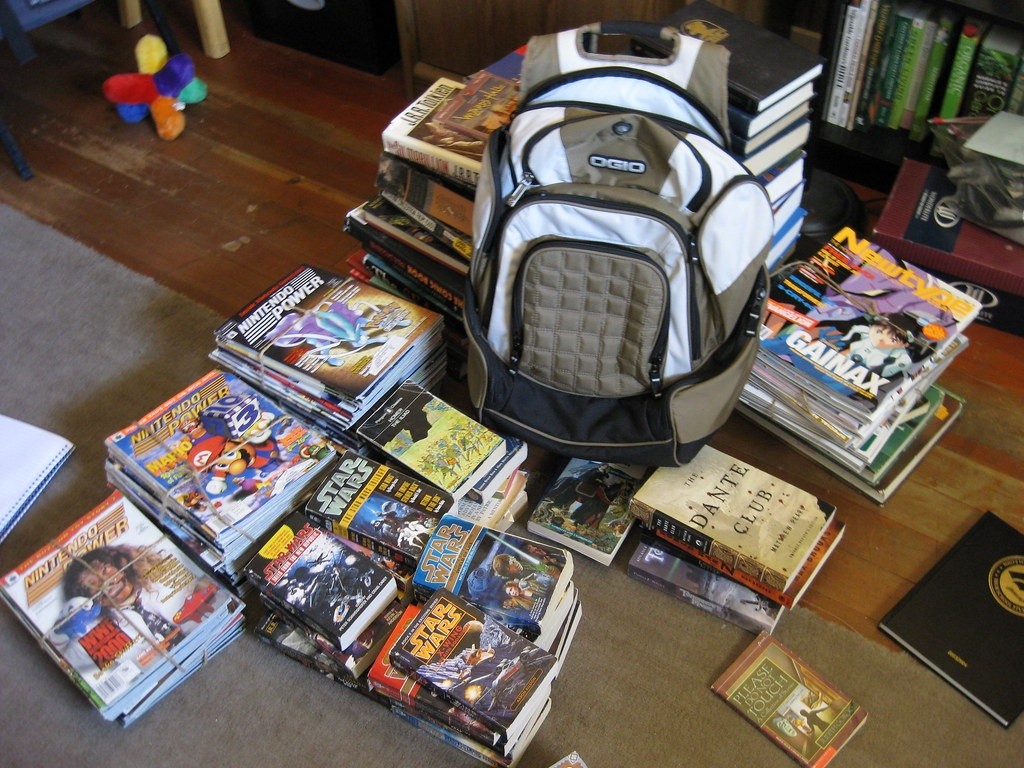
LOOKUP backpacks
[463,20,775,469]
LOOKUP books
[0,1,1024,768]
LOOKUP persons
[416,122,484,156]
[47,545,217,672]
[501,581,546,611]
[800,704,832,734]
[491,554,558,597]
[771,715,797,737]
[498,598,535,615]
[521,544,564,572]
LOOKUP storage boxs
[873,157,1024,338]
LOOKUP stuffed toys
[103,34,207,140]
